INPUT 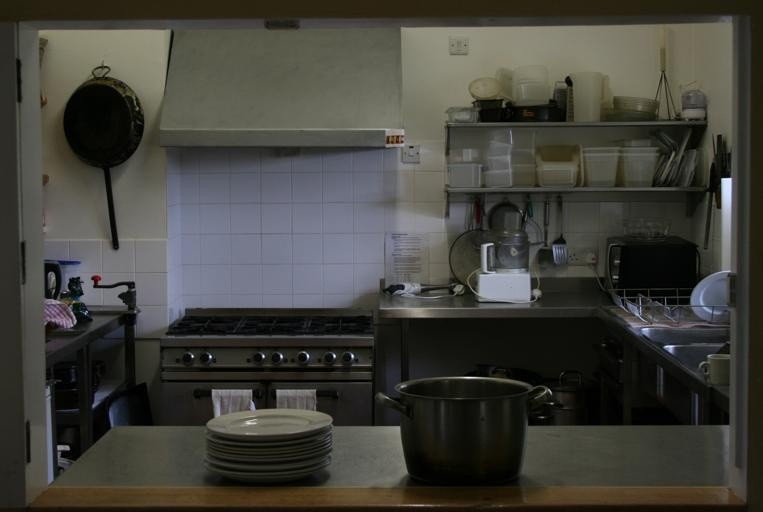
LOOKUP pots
[63,66,145,251]
[374,376,552,481]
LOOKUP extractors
[156,27,406,149]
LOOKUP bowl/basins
[613,95,660,113]
[468,77,502,100]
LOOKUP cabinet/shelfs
[44,281,137,469]
[443,121,708,287]
[600,298,733,422]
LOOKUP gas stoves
[158,315,373,370]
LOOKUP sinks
[626,325,732,382]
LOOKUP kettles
[569,71,609,123]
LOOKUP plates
[689,269,732,325]
[199,408,334,484]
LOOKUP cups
[696,353,730,385]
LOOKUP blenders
[475,210,531,304]
